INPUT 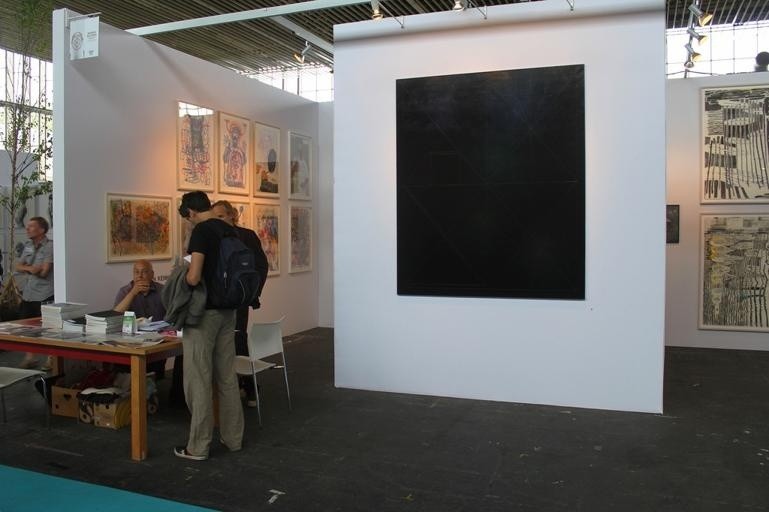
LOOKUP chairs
[0,365,47,438]
[236,315,293,425]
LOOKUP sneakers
[248,401,257,407]
[219,437,241,451]
[239,388,246,397]
[174,446,208,461]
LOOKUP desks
[0,316,183,462]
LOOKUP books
[41,303,170,333]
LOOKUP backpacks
[208,237,260,308]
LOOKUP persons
[12,215,57,371]
[173,187,245,461]
[211,200,260,407]
[114,260,171,323]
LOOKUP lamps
[683,0,713,79]
[452,0,488,20]
[371,0,404,29]
[293,42,312,65]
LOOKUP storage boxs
[51,384,131,430]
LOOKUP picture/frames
[701,85,769,205]
[698,211,769,332]
[667,204,679,245]
[108,102,313,278]
